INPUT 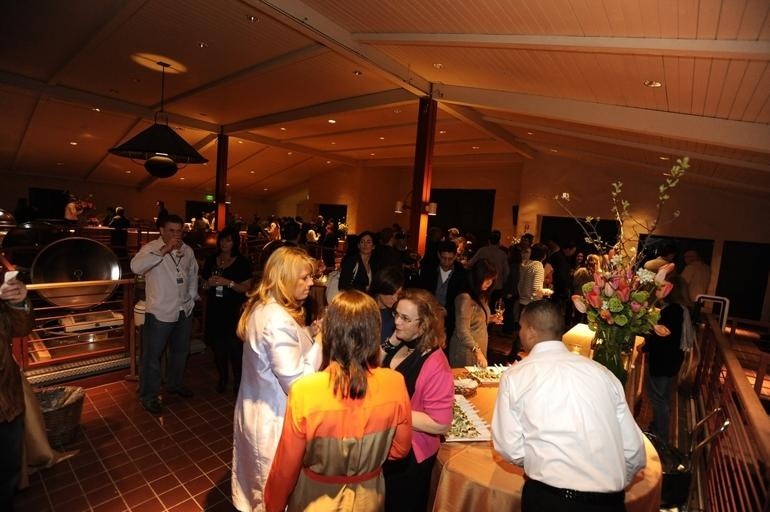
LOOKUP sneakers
[175,384,194,398]
[141,392,163,415]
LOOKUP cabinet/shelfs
[426,367,664,512]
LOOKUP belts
[524,477,626,504]
[303,465,383,485]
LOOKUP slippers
[25,448,80,476]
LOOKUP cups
[211,270,222,279]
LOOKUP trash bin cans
[32,385,85,449]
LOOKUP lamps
[108,61,208,178]
[225,195,232,205]
[421,202,437,215]
[394,201,411,213]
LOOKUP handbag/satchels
[678,302,702,391]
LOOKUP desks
[563,323,644,415]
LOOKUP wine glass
[174,238,186,255]
[316,305,330,332]
[493,302,507,325]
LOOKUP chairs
[668,345,706,447]
[646,409,729,507]
[310,286,328,321]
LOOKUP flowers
[552,156,690,381]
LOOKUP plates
[544,289,555,296]
[465,364,510,385]
[441,394,492,444]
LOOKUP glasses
[359,240,373,246]
[390,310,423,325]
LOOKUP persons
[229,215,712,510]
[0,195,289,512]
